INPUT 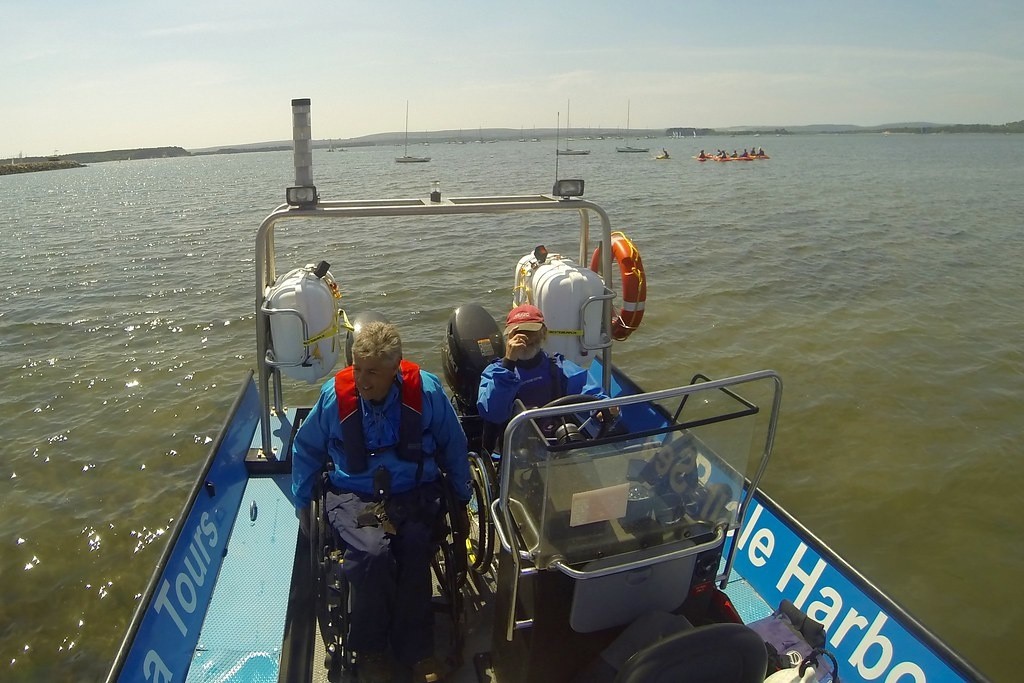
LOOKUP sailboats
[614,98,650,153]
[394,100,433,162]
[557,98,591,155]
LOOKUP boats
[694,152,770,162]
[654,155,669,159]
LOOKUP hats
[502,305,546,335]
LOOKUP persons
[698,150,705,158]
[757,147,764,155]
[731,150,737,157]
[476,304,623,458]
[739,148,748,157]
[717,149,722,156]
[722,151,726,158]
[750,147,755,155]
[292,321,474,683]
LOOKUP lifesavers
[590,228,649,342]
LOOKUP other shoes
[361,654,394,683]
[410,656,445,683]
